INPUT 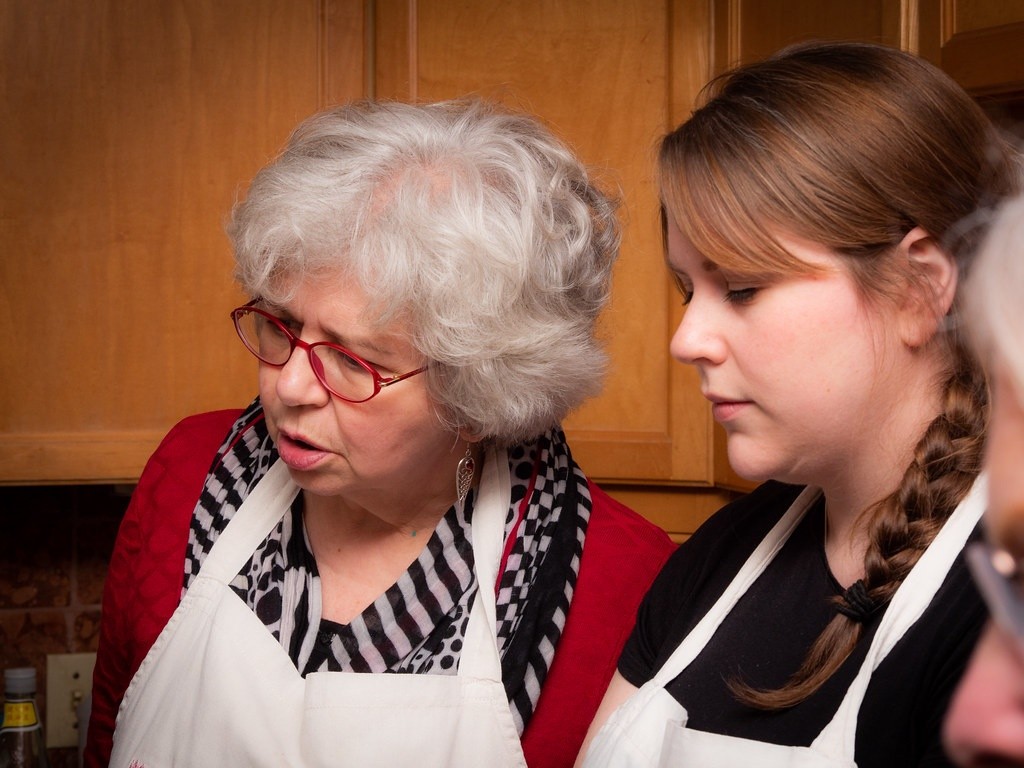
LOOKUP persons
[944,195,1024,768]
[82,100,681,768]
[527,42,1024,768]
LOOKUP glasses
[230,295,443,403]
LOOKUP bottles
[0,667,48,768]
